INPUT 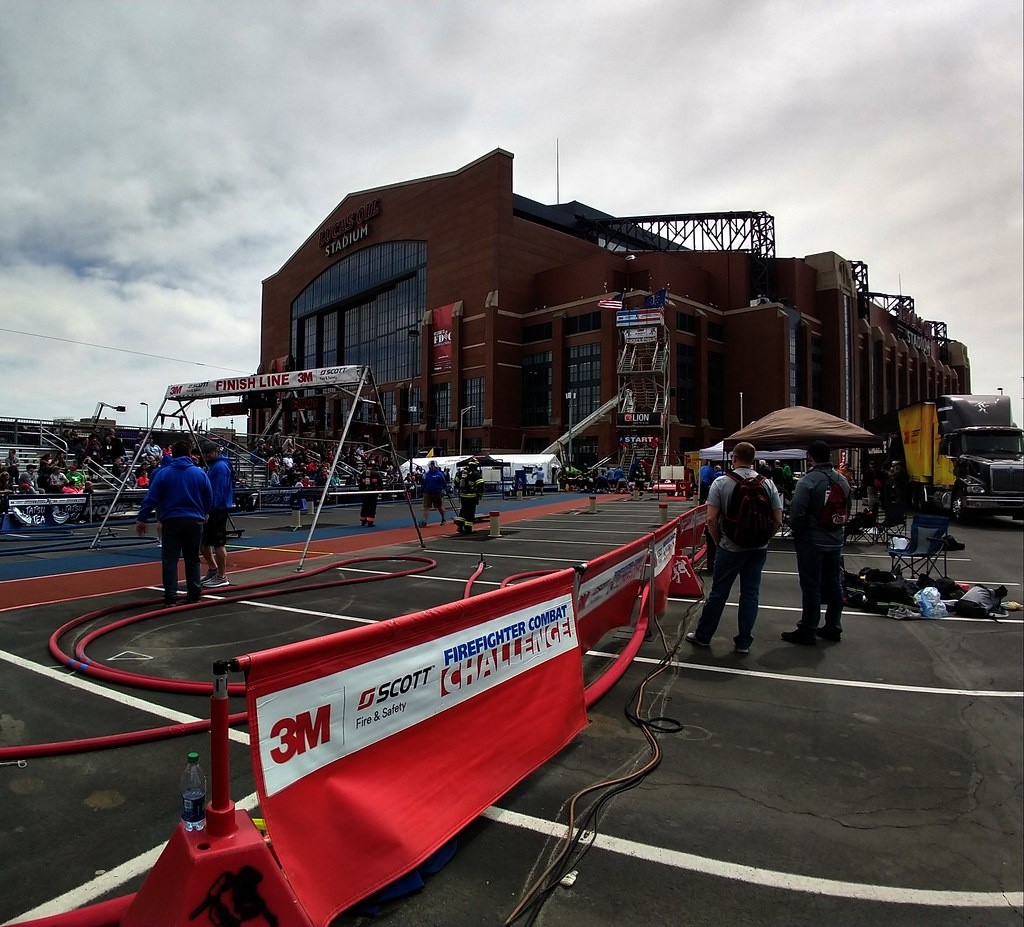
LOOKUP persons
[0,429,204,494]
[403,458,485,535]
[135,439,211,608]
[201,442,233,587]
[780,440,853,646]
[686,442,783,653]
[150,455,173,548]
[606,466,623,494]
[629,460,643,480]
[532,467,544,496]
[251,429,400,528]
[833,463,849,473]
[758,460,795,494]
[558,467,567,491]
[700,460,723,503]
[860,461,906,510]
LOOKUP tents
[698,421,806,505]
[456,450,511,500]
[400,454,562,486]
[722,406,883,517]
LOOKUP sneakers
[199,571,229,587]
[183,594,201,603]
[893,608,921,620]
[685,631,711,647]
[732,639,748,653]
[887,607,900,618]
[166,598,176,607]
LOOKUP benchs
[0,446,389,494]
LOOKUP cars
[792,472,805,480]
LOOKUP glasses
[806,452,810,457]
[730,454,737,459]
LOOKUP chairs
[842,500,951,581]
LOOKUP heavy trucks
[860,395,1024,524]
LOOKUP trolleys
[448,498,490,523]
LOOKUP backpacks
[723,471,773,548]
[811,469,850,533]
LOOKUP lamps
[625,255,636,262]
[408,329,422,337]
[750,300,759,308]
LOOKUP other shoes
[157,540,162,548]
[457,527,463,533]
[782,629,816,645]
[816,627,841,641]
[359,520,376,528]
[440,520,446,525]
[466,529,472,533]
[419,522,426,527]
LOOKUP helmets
[367,460,376,467]
[469,458,479,466]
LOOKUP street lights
[140,402,148,432]
[566,389,576,454]
[460,406,476,456]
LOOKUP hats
[201,441,219,454]
[68,466,76,471]
[25,464,37,471]
[758,460,766,467]
[775,460,780,463]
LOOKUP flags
[597,293,624,310]
[644,289,667,308]
